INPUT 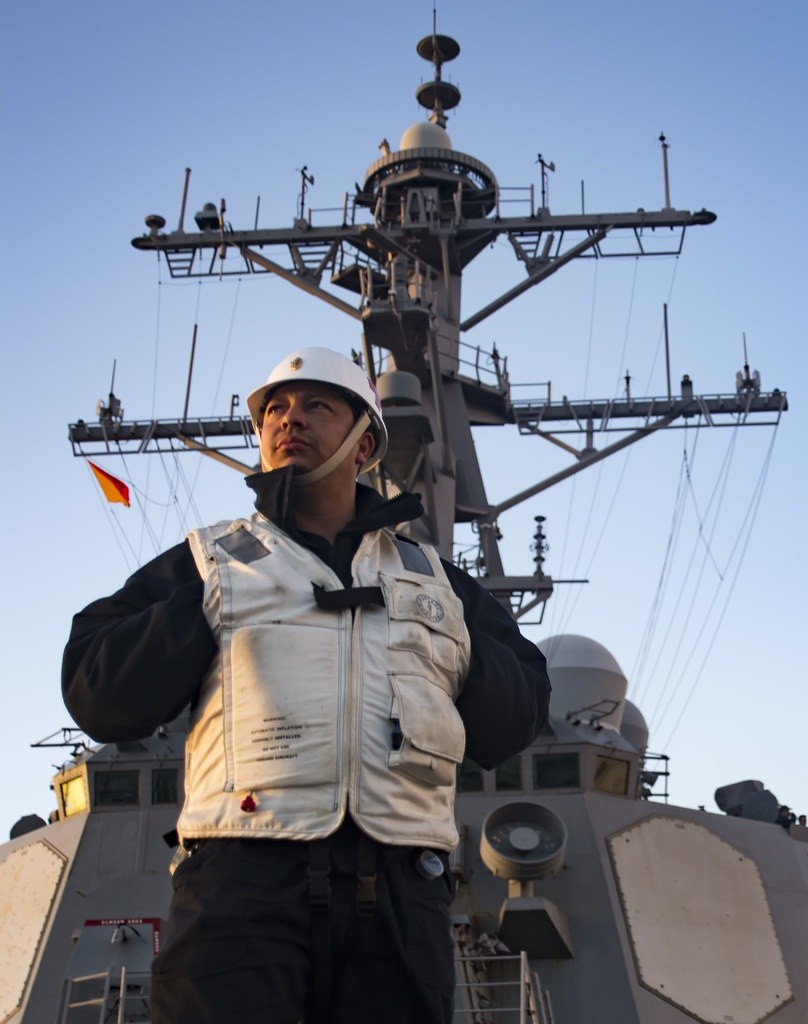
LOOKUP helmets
[246,348,388,476]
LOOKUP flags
[88,460,130,508]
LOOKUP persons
[60,346,554,1024]
